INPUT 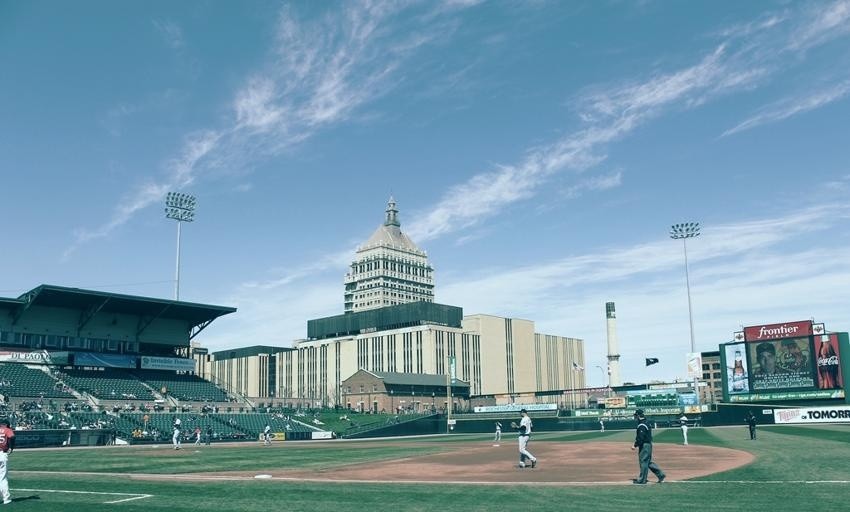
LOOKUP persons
[679,412,688,445]
[267,406,355,430]
[495,420,503,441]
[631,410,666,484]
[369,404,446,425]
[744,411,756,439]
[753,342,793,380]
[511,408,536,468]
[0,419,15,504]
[600,420,605,432]
[264,423,272,446]
[109,397,237,449]
[0,385,115,429]
[121,392,137,398]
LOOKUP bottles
[819,334,838,390]
[732,350,746,392]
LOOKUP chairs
[0,362,325,444]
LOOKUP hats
[634,410,643,416]
[756,343,774,354]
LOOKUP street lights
[163,190,198,301]
[390,389,394,413]
[342,387,348,409]
[368,389,371,410]
[411,391,415,409]
[280,384,285,407]
[270,389,274,402]
[452,393,455,409]
[311,387,315,409]
[431,391,436,404]
[596,365,606,397]
[668,220,701,353]
